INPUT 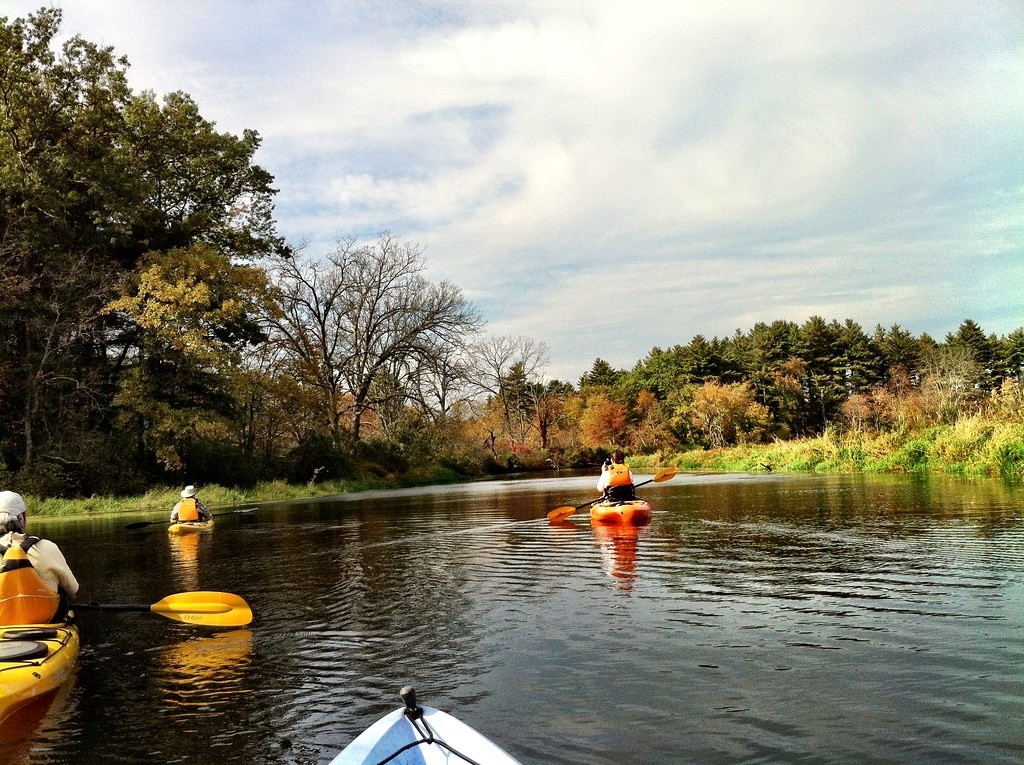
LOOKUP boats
[167,518,216,534]
[591,500,652,525]
[327,685,524,765]
[0,622,81,725]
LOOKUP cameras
[605,458,612,465]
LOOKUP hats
[611,450,624,463]
[181,485,199,497]
[0,491,26,521]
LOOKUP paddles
[546,466,676,523]
[63,590,255,628]
[126,507,259,531]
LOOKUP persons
[597,449,635,502]
[0,490,79,625]
[170,485,213,524]
